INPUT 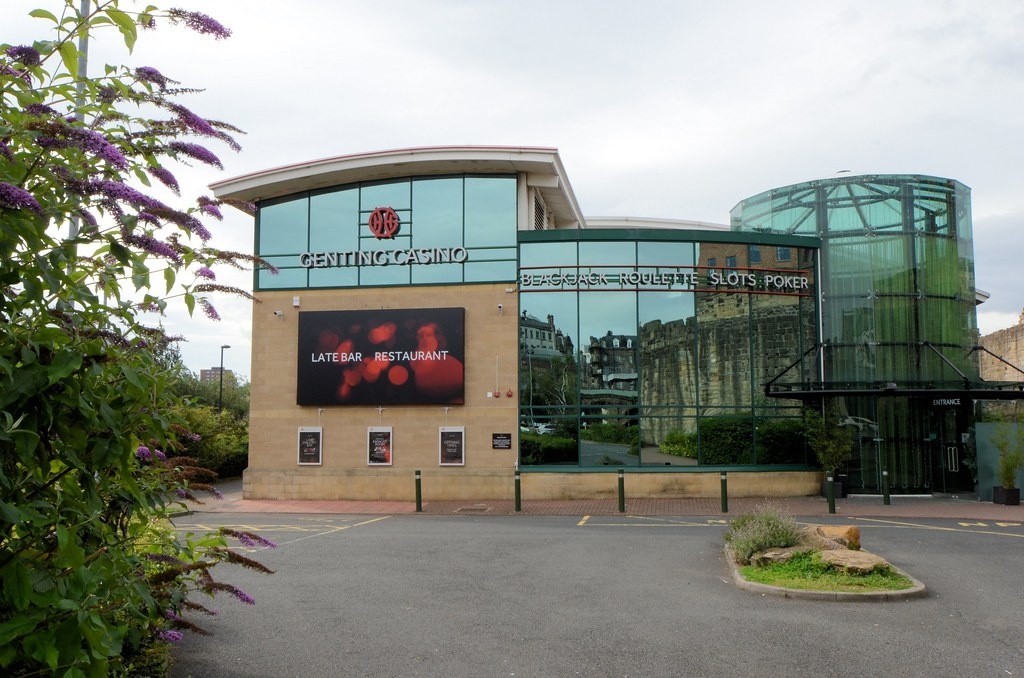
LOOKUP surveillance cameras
[497,304,503,310]
[273,310,283,317]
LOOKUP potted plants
[983,404,1024,506]
[798,409,861,498]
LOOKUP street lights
[219,344,231,412]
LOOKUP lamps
[883,383,897,392]
[293,296,300,308]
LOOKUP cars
[520,417,577,440]
